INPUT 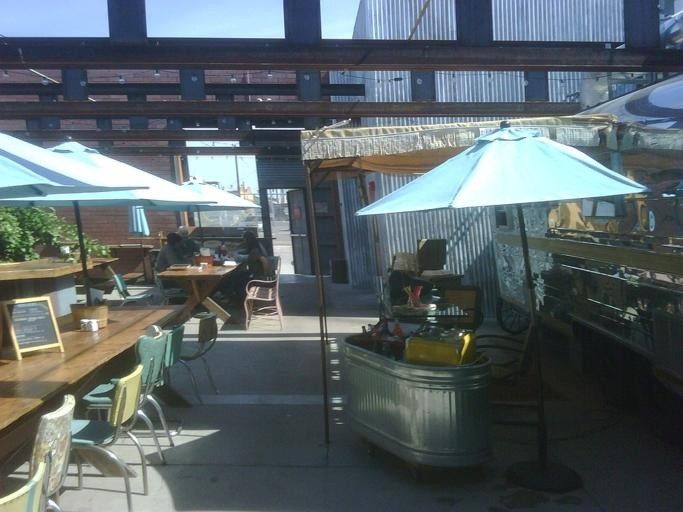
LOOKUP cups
[87,320,98,331]
[201,263,208,270]
[80,318,88,330]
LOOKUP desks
[0,257,121,324]
[158,261,242,324]
[0,303,188,488]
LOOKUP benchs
[243,256,284,330]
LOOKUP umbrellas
[0,133,149,199]
[0,136,218,307]
[135,177,261,237]
[353,121,655,469]
[128,205,150,284]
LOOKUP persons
[153,225,271,304]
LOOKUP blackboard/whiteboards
[1,296,62,353]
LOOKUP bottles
[361,324,405,361]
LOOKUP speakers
[330,258,348,284]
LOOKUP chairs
[177,315,219,405]
[0,462,48,512]
[78,333,174,466]
[143,255,153,284]
[155,326,184,392]
[112,273,153,307]
[1,394,76,512]
[69,364,148,512]
